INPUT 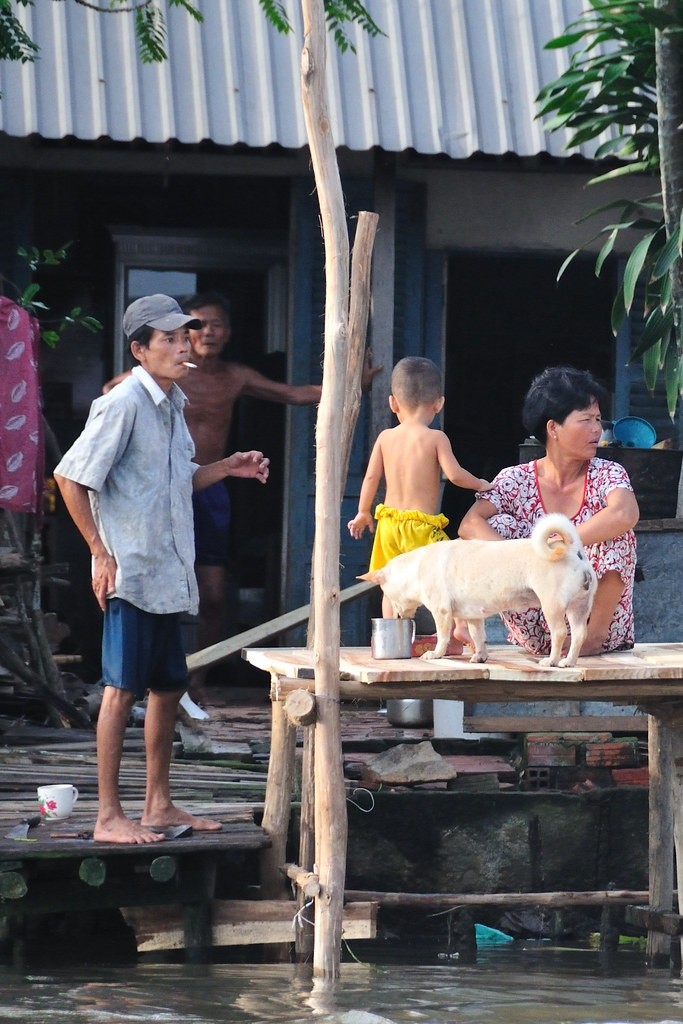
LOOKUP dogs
[355,513,598,667]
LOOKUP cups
[370,616,416,660]
[37,784,78,821]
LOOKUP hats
[122,294,202,340]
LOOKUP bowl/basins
[385,699,433,728]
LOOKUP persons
[347,356,496,654]
[458,366,640,656]
[54,293,270,845]
[102,296,384,707]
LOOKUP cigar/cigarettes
[182,361,198,369]
